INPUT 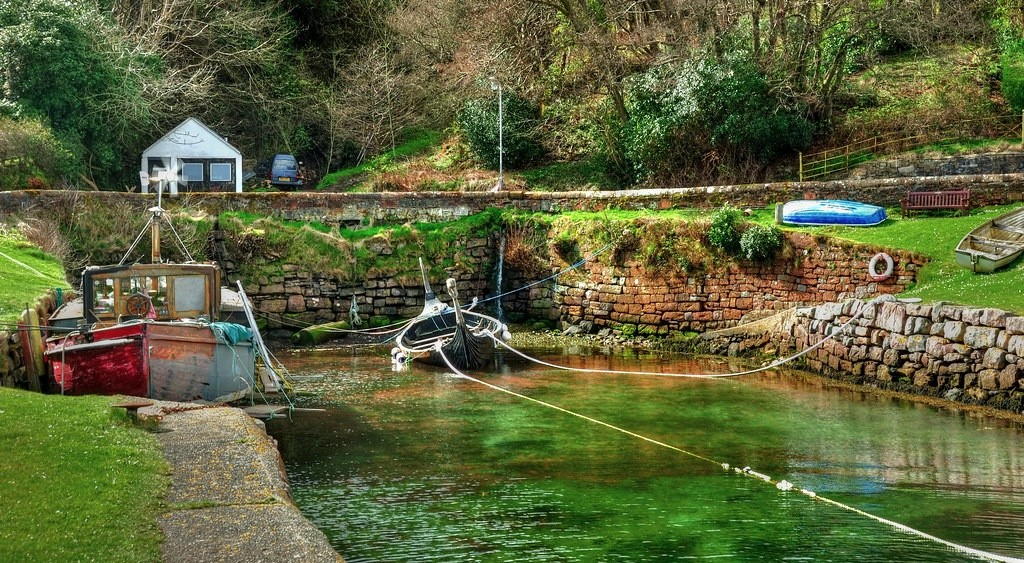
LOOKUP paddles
[236,278,282,393]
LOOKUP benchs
[899,190,970,218]
[990,223,1024,234]
[971,237,1024,250]
[404,318,483,345]
[412,320,493,349]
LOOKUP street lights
[489,76,502,192]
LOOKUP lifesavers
[868,252,895,282]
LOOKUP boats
[396,256,502,371]
[782,199,889,227]
[45,207,256,405]
[955,207,1024,275]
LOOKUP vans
[257,156,301,192]
